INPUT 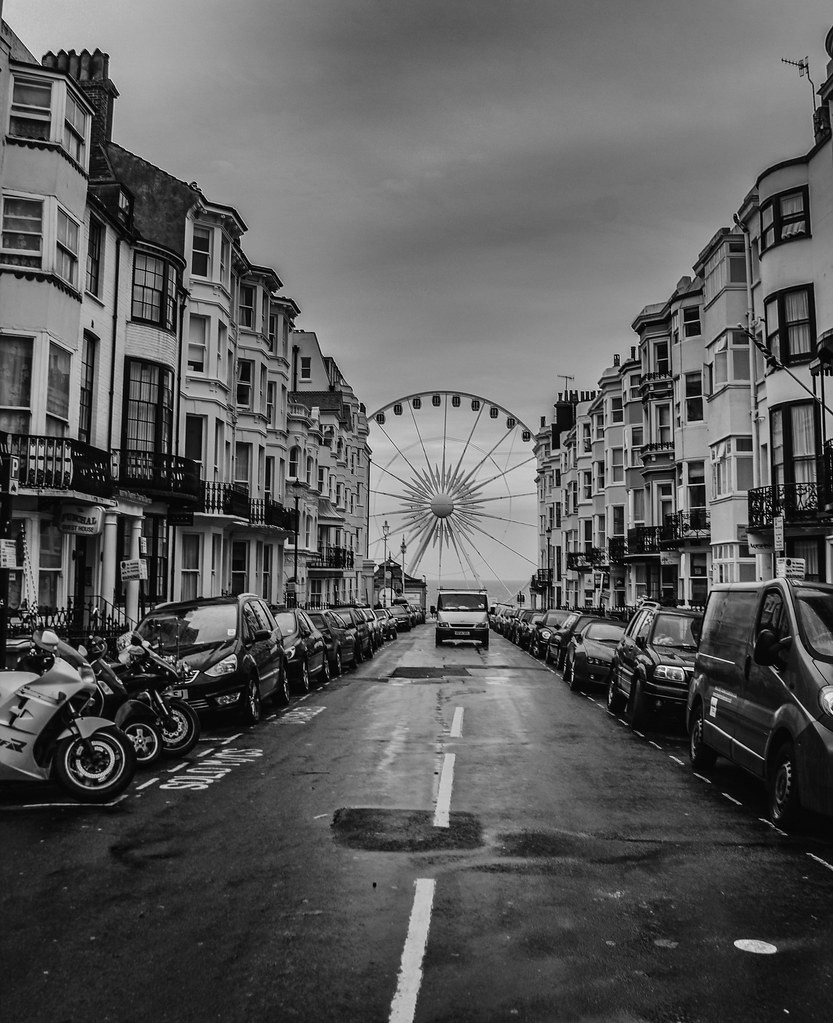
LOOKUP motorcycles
[0,628,202,804]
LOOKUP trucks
[430,586,495,649]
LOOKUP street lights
[400,534,407,594]
[381,520,390,609]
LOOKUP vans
[684,576,832,834]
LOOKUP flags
[744,329,783,370]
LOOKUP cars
[332,604,425,663]
[269,607,331,697]
[488,602,620,671]
[304,610,356,678]
[562,620,628,694]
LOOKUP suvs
[607,601,707,730]
[130,592,289,727]
